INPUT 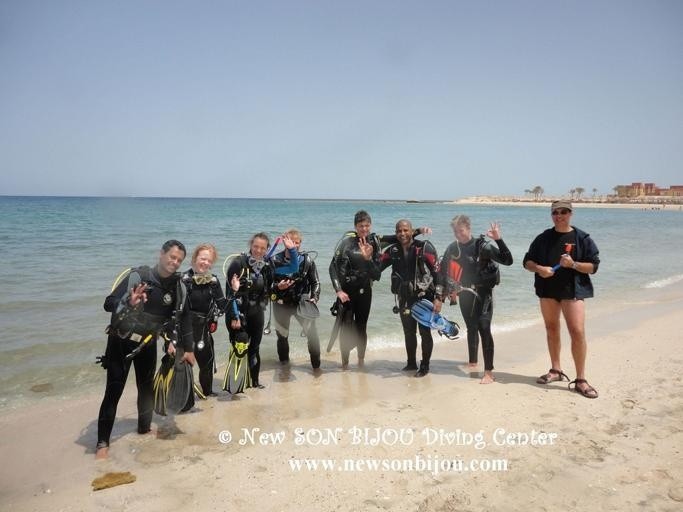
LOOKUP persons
[329,209,432,371]
[268,230,326,373]
[357,219,444,378]
[161,244,241,413]
[95,239,196,459]
[522,199,600,398]
[225,233,295,390]
[440,215,513,385]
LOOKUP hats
[551,200,572,212]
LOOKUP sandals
[568,379,598,398]
[536,369,570,384]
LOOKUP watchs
[570,262,576,269]
